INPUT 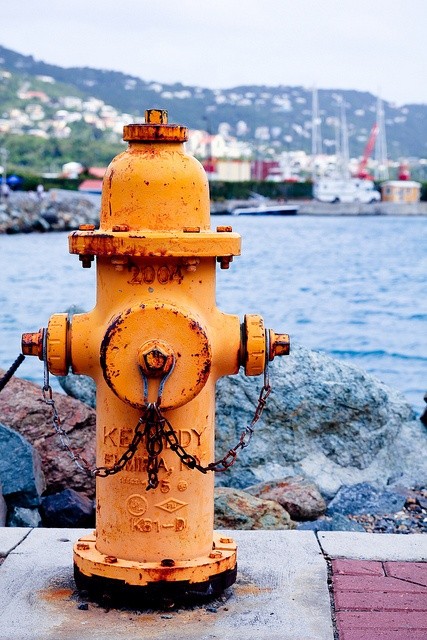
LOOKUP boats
[233,204,301,216]
